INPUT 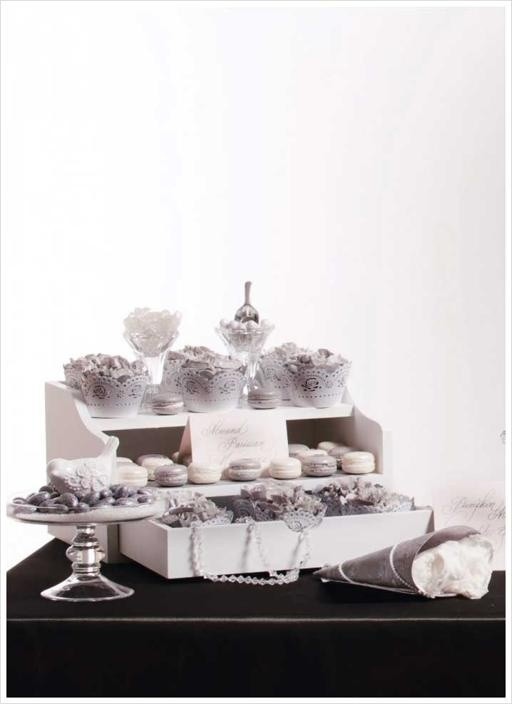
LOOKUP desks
[7,537,506,697]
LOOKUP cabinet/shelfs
[46,375,439,581]
